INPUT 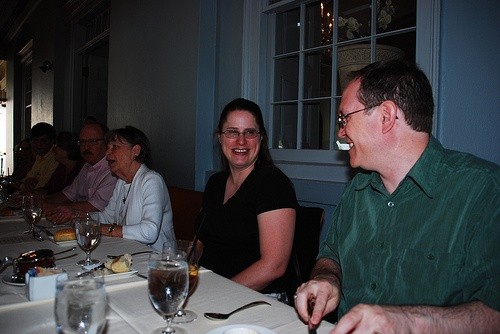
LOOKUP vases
[336,46,404,93]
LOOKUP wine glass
[72,212,102,265]
[146,250,190,334]
[160,239,198,323]
[22,194,43,234]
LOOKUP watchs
[107,223,117,237]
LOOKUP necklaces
[122,181,132,204]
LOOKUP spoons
[107,250,154,259]
[204,300,271,320]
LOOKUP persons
[292,58,500,334]
[46,125,178,257]
[16,121,59,191]
[193,97,301,308]
[38,122,120,224]
[25,131,85,198]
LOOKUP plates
[3,278,26,286]
[83,263,138,281]
[47,235,78,246]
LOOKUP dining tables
[0,174,335,334]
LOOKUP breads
[105,253,133,273]
[54,228,76,242]
[0,208,14,216]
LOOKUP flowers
[335,0,394,47]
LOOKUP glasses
[78,139,105,145]
[55,144,59,147]
[336,103,399,128]
[220,129,260,139]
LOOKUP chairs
[278,205,325,306]
[167,186,204,252]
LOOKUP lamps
[40,61,54,72]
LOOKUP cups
[53,272,109,334]
